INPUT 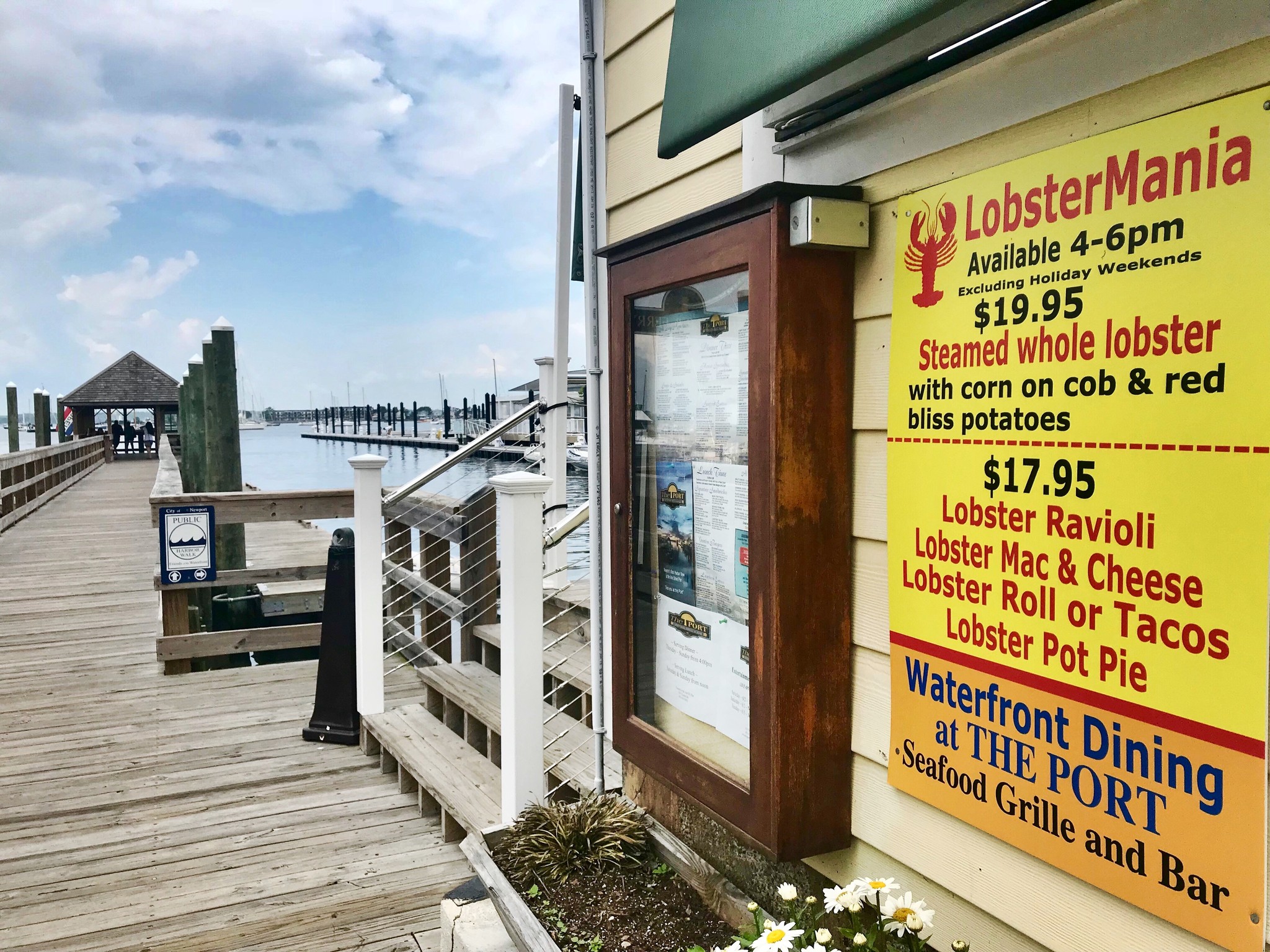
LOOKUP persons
[140,422,155,459]
[28,423,31,429]
[124,421,136,454]
[381,427,382,434]
[95,427,105,436]
[112,420,123,455]
[383,425,392,435]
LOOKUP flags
[56,407,73,435]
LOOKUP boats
[524,442,588,471]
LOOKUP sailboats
[4,398,177,432]
[297,359,498,429]
[234,340,280,430]
